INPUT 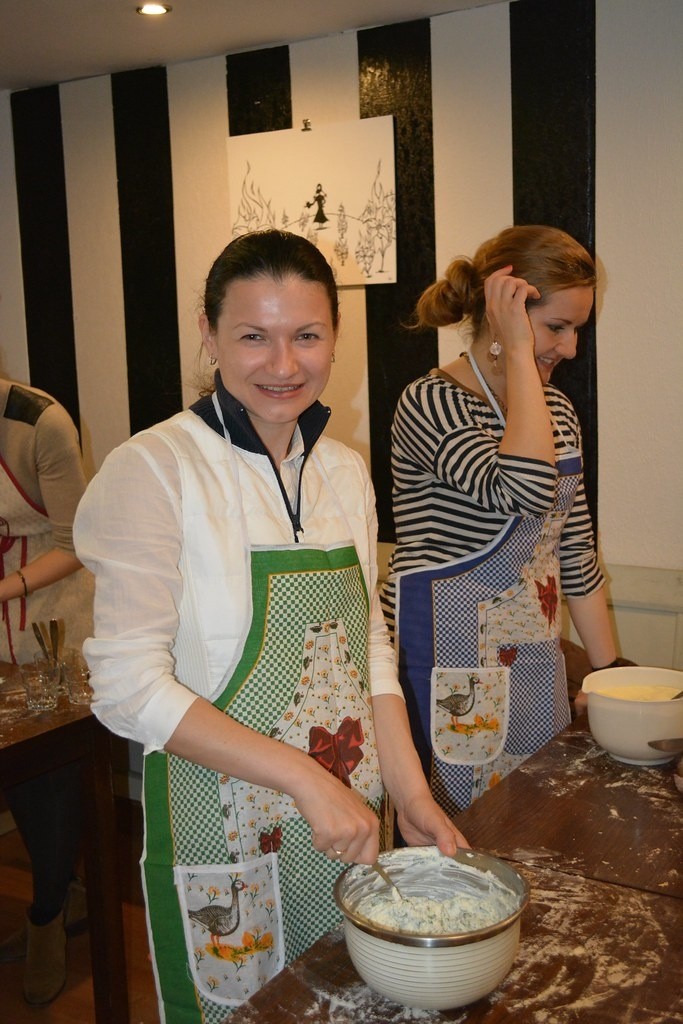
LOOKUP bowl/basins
[333,844,530,1010]
[581,666,683,765]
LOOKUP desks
[0,663,137,1024]
[215,714,683,1024]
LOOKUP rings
[331,844,347,855]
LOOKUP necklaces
[459,351,509,417]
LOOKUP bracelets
[16,570,27,597]
[591,660,619,673]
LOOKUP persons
[0,374,91,1004]
[72,229,476,1023]
[375,223,620,852]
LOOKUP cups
[34,648,76,695]
[18,660,58,710]
[62,655,93,705]
